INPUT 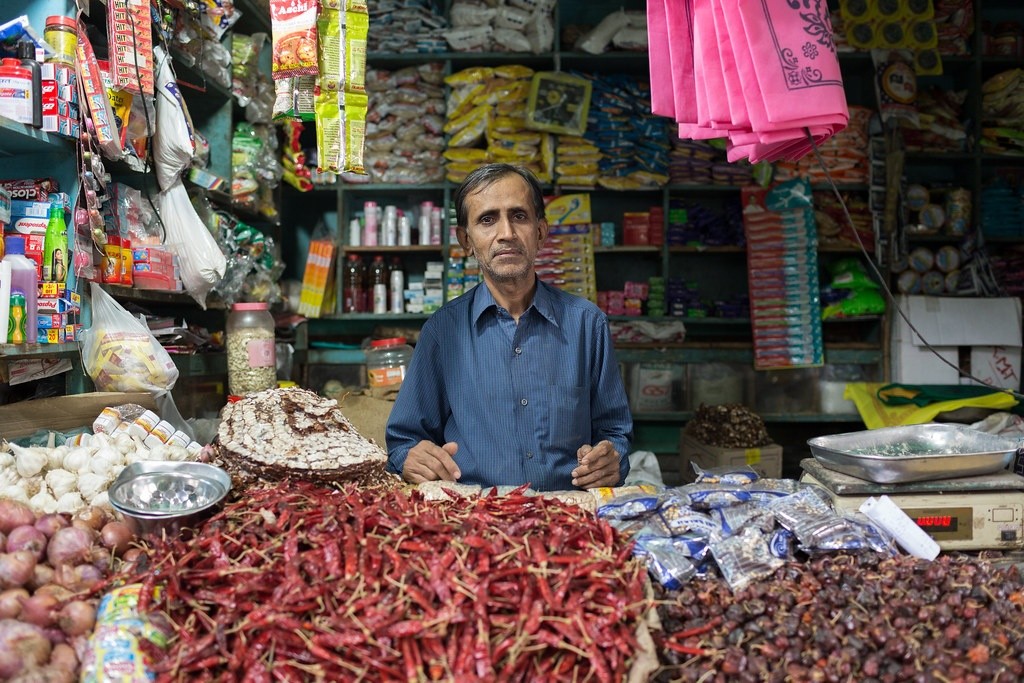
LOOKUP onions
[0,498,150,683]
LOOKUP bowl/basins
[108,460,231,537]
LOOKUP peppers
[48,480,1023,683]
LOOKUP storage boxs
[333,387,396,447]
[680,439,782,486]
[891,296,1022,394]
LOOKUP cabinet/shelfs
[0,1,282,356]
[288,1,1024,422]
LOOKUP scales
[800,455,1024,555]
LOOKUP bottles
[344,255,405,313]
[225,302,276,395]
[1,236,38,342]
[7,289,28,344]
[43,208,69,282]
[367,336,414,389]
[0,41,43,129]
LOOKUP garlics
[0,430,194,517]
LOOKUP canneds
[99,233,133,288]
[43,16,79,71]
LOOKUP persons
[385,162,634,492]
